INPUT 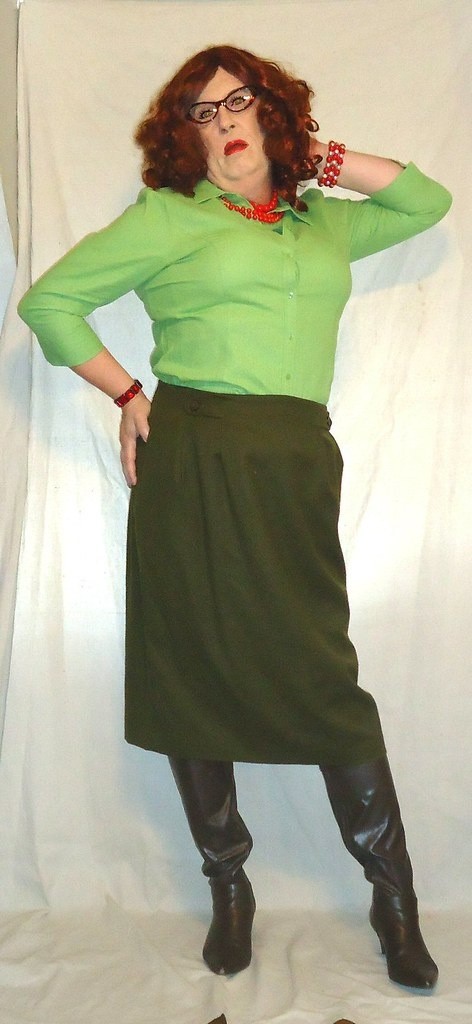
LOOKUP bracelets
[114,379,143,408]
[320,139,347,187]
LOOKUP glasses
[187,83,258,125]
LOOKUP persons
[18,43,440,990]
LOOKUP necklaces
[208,181,286,223]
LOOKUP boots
[160,755,256,975]
[314,756,439,991]
